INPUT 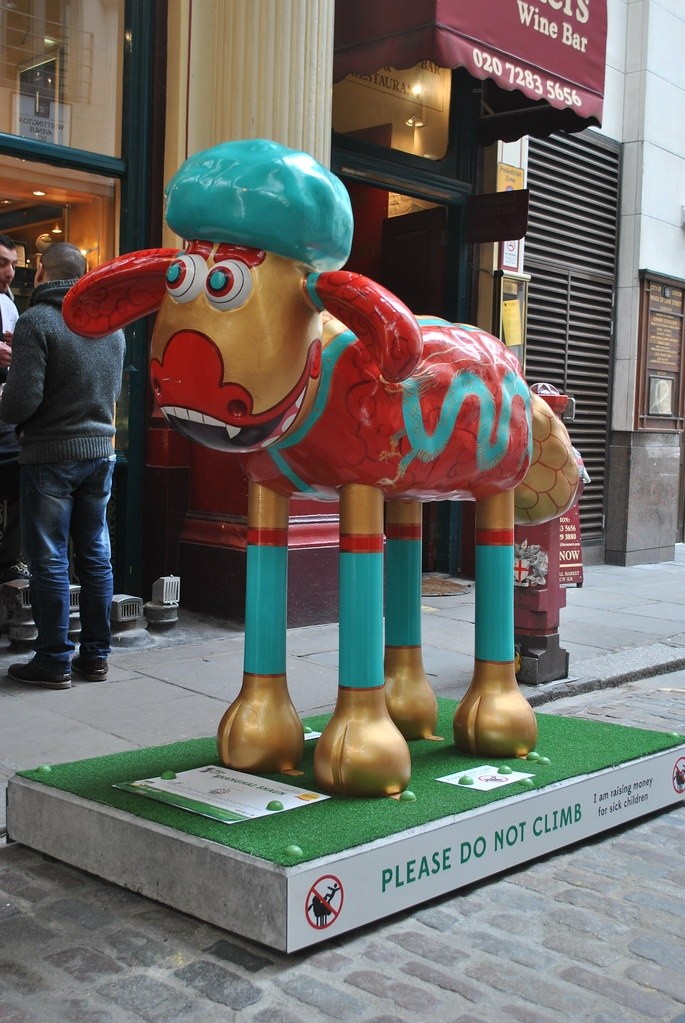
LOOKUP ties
[0,309,9,384]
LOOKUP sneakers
[73,655,108,682]
[7,660,71,689]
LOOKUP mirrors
[15,49,60,144]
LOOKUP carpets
[421,574,471,596]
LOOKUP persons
[0,233,128,688]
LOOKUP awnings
[334,0,609,147]
